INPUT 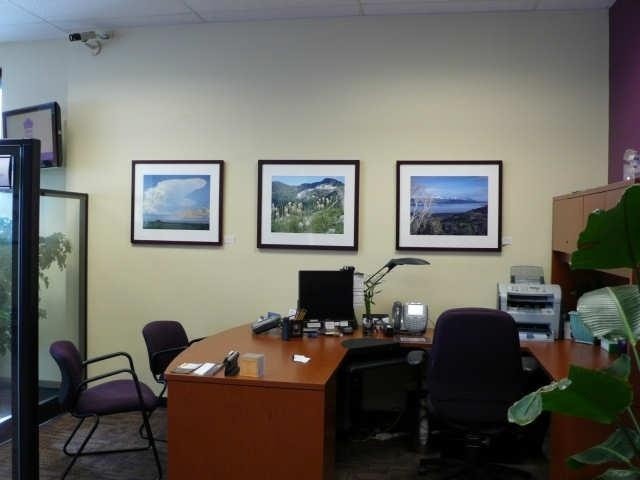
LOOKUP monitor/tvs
[3,102,63,168]
[299,271,354,336]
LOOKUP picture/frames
[131,159,224,244]
[256,159,360,250]
[396,159,503,252]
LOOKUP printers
[497,266,562,342]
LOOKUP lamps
[363,257,431,330]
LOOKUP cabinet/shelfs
[550,176,640,366]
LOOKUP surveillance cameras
[69,30,96,42]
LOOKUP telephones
[391,300,428,336]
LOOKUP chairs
[419,308,548,479]
[137,319,211,444]
[49,340,167,479]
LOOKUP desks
[163,319,640,479]
